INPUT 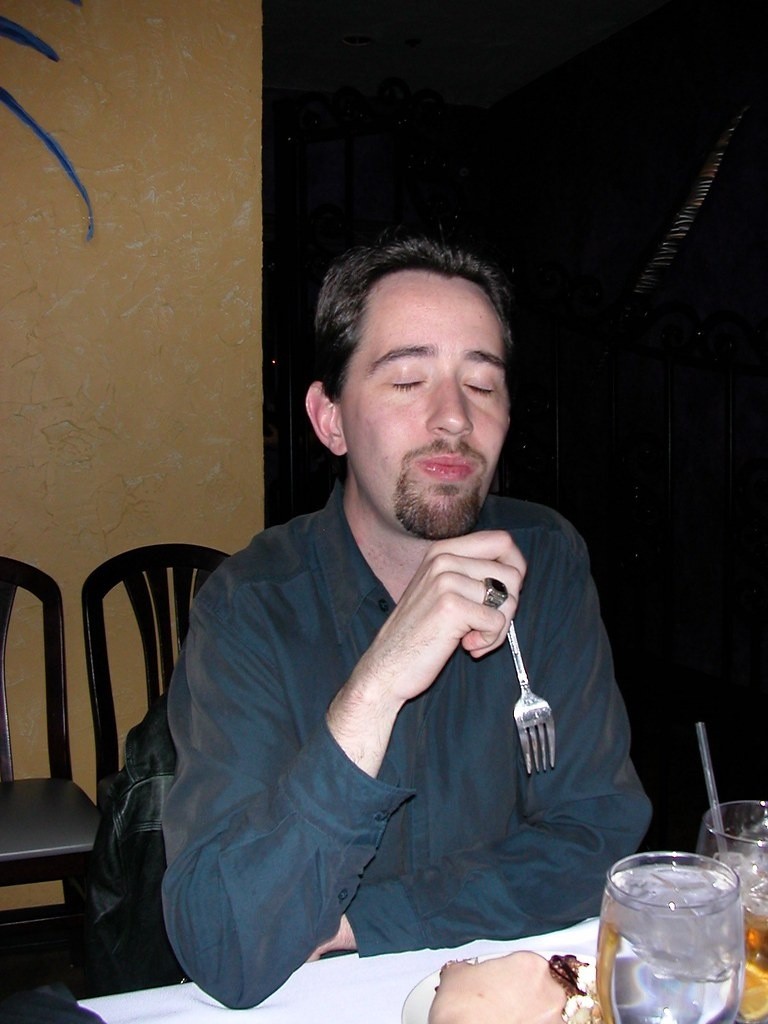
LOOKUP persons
[159,236,650,1013]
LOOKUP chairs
[0,542,233,1006]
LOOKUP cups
[595,851,748,1024]
[694,799,768,1024]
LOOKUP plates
[399,951,598,1024]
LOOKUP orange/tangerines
[738,962,768,1020]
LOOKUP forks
[507,619,557,774]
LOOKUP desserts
[427,950,601,1024]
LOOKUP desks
[76,916,601,1024]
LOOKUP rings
[484,578,509,607]
